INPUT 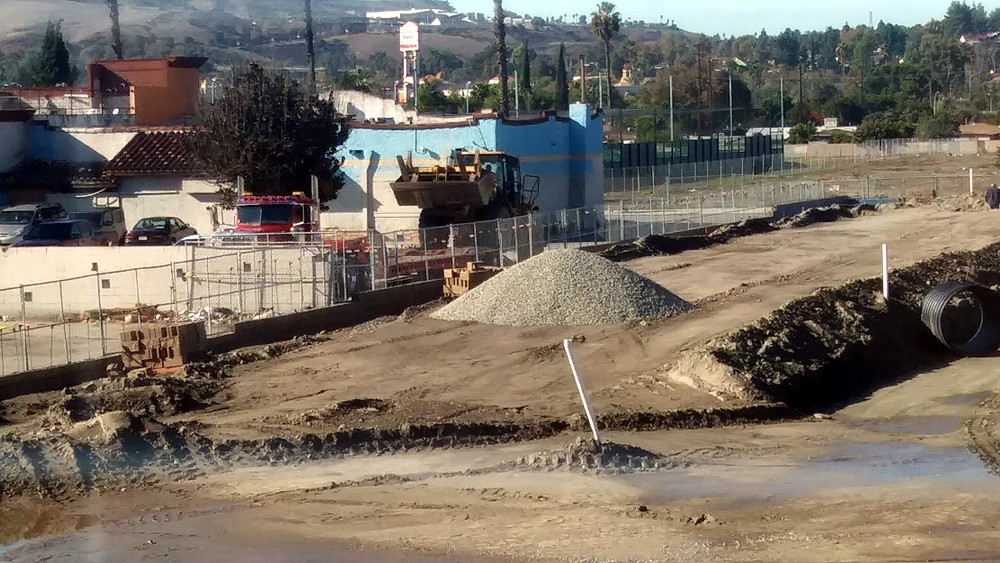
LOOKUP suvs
[0,200,68,246]
[61,206,127,246]
[10,216,99,248]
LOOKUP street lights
[466,80,471,114]
[714,68,733,138]
[584,62,602,109]
[767,69,784,133]
[655,65,674,141]
[393,80,400,104]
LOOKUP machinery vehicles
[389,147,542,251]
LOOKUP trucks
[231,174,321,248]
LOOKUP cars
[3,82,23,88]
[123,217,200,247]
